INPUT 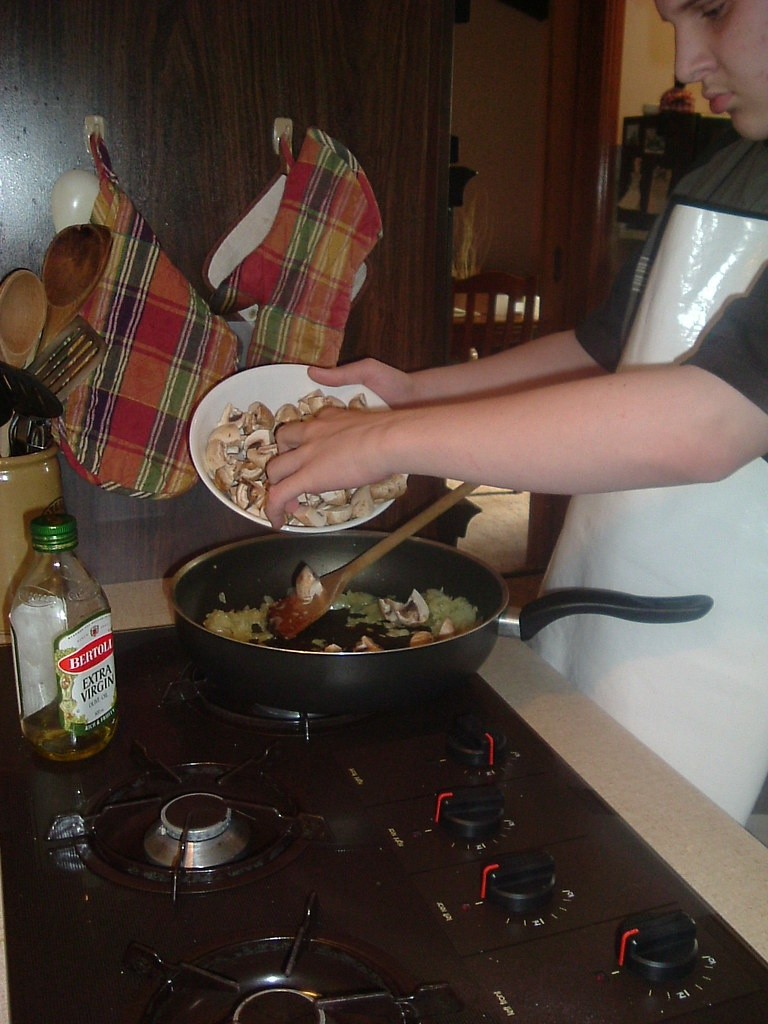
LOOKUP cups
[0,441,63,642]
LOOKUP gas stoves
[0,624,768,1024]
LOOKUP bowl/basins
[642,103,659,114]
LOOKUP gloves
[208,127,384,372]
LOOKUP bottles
[9,511,120,761]
[659,77,696,114]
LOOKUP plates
[189,361,411,533]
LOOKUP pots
[166,527,713,718]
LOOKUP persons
[659,78,695,112]
[264,0,768,828]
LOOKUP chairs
[451,270,540,362]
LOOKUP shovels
[267,477,484,644]
[0,360,64,455]
[21,313,109,417]
